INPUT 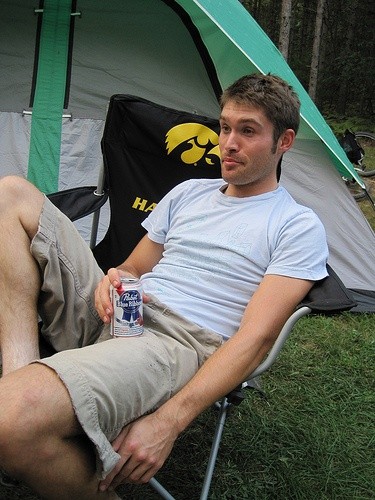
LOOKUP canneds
[109,278,144,337]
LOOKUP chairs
[46,94,358,500]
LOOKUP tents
[0,0,375,316]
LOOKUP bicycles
[337,131,375,201]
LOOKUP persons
[0,74,329,500]
